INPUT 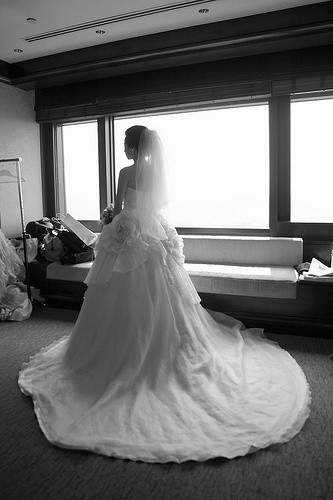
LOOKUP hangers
[0,159,27,184]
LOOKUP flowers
[100,204,113,225]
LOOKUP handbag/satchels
[56,230,96,265]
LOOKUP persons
[65,125,212,394]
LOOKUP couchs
[42,231,333,339]
